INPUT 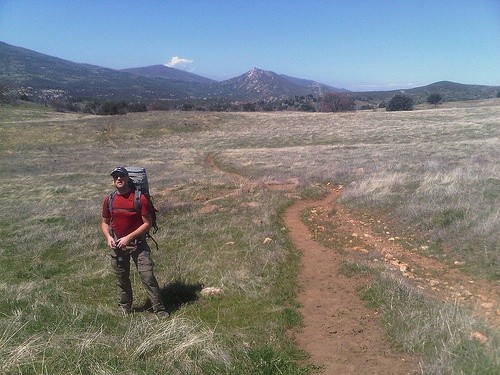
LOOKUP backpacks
[108,166,157,228]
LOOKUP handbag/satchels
[113,234,142,255]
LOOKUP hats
[110,167,128,177]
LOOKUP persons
[102,167,169,321]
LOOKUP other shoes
[155,310,169,319]
[117,303,132,314]
